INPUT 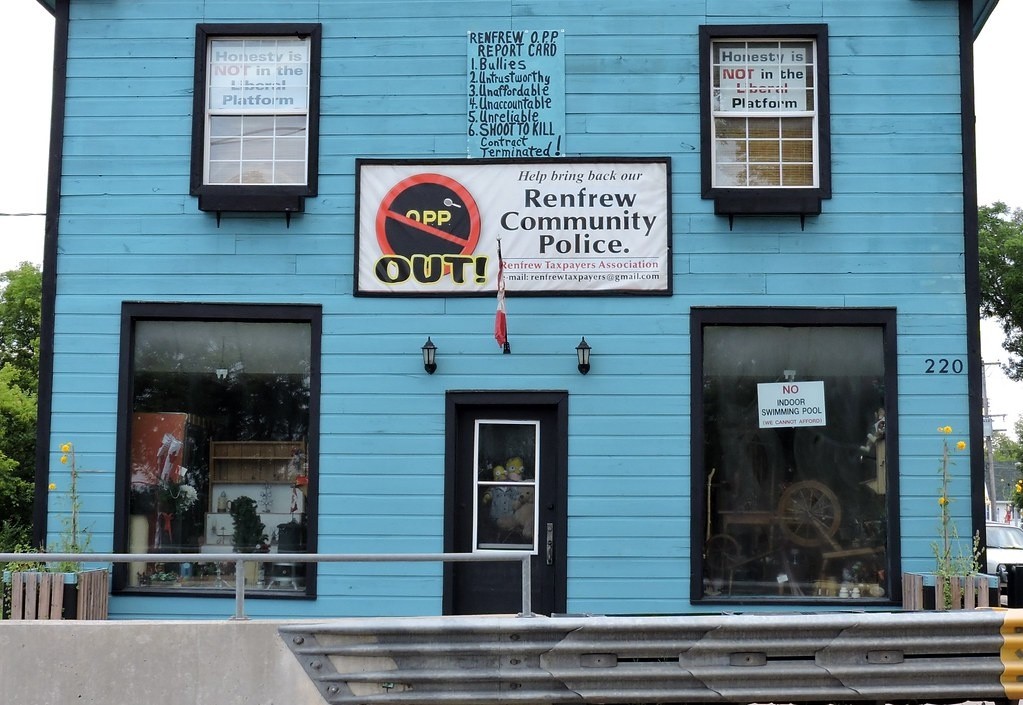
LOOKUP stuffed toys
[482,456,534,545]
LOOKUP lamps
[421,337,437,375]
[575,337,591,374]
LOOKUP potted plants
[229,495,269,585]
[128,487,158,587]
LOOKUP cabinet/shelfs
[198,440,312,567]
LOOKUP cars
[984,520,1023,581]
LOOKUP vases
[3,566,107,618]
[911,569,1001,609]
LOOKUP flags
[495,257,507,347]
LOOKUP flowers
[5,443,98,573]
[930,427,966,573]
